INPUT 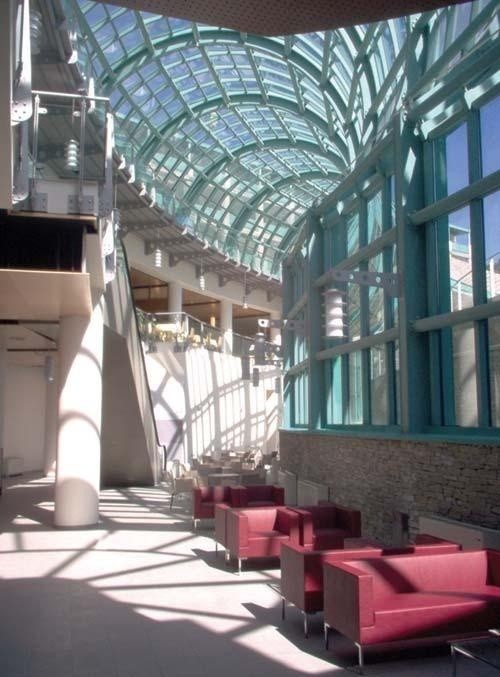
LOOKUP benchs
[191,482,362,577]
[279,533,500,677]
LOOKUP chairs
[168,446,267,512]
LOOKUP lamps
[321,270,402,340]
[64,98,80,170]
[199,255,206,293]
[153,225,163,268]
[241,272,250,312]
[254,318,312,365]
